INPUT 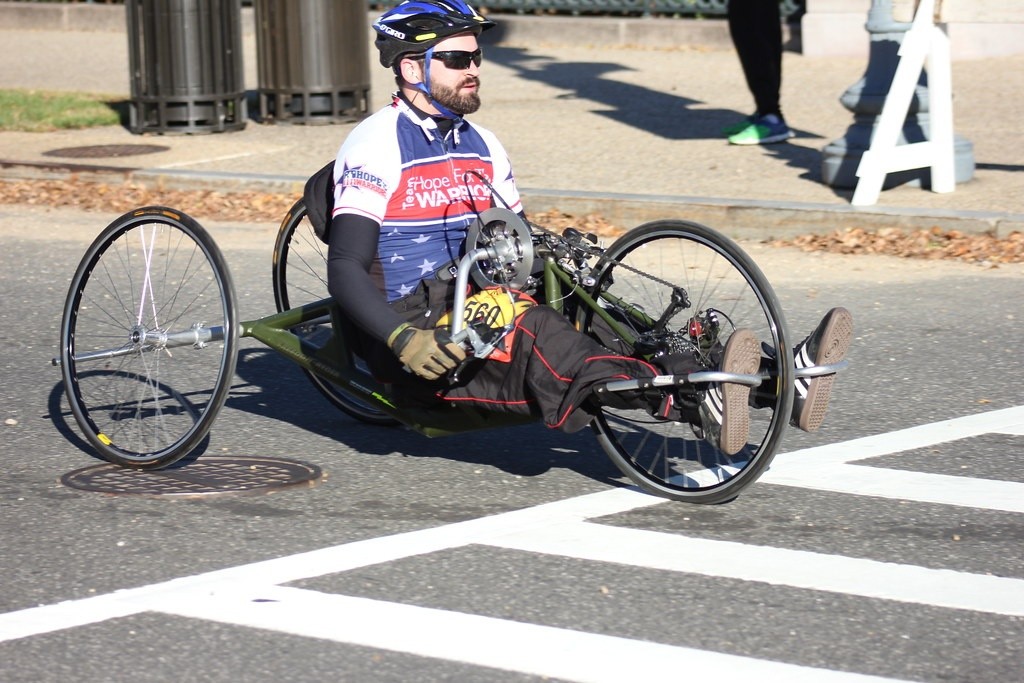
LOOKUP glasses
[403,48,483,70]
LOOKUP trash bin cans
[252,0,375,127]
[121,0,246,137]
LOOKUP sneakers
[720,112,760,136]
[729,116,791,144]
[762,306,853,431]
[698,327,762,455]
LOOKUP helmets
[372,0,497,69]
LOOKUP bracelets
[386,322,413,349]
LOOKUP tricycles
[49,157,851,505]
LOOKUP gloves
[389,326,467,380]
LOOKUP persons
[326,0,854,455]
[724,0,793,144]
